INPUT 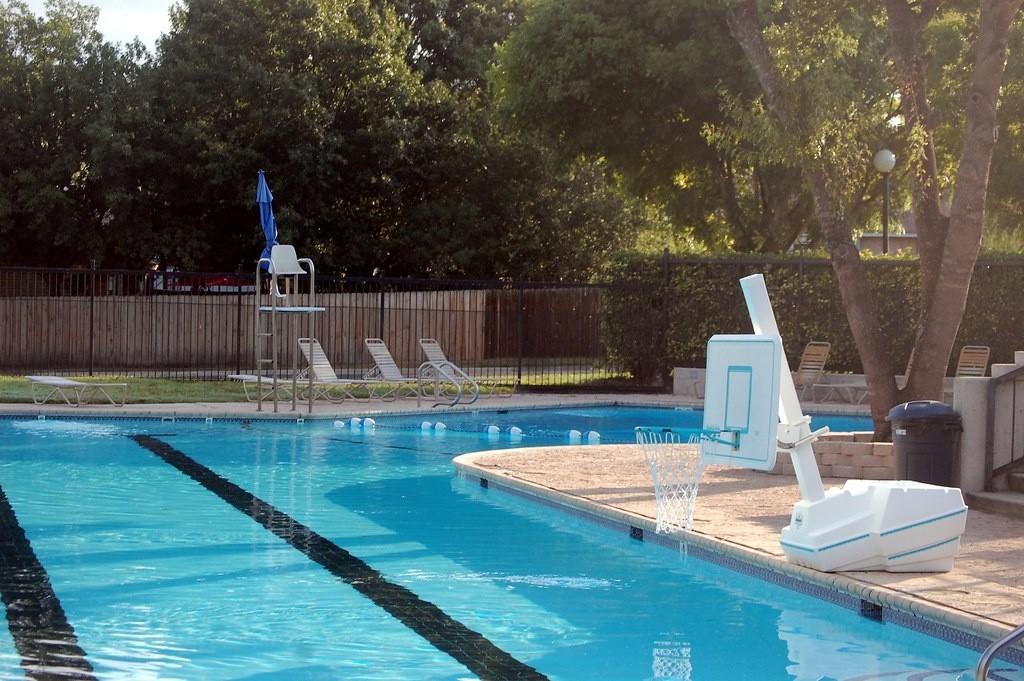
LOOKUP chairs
[296,338,403,405]
[813,383,868,404]
[362,338,467,401]
[230,374,350,404]
[25,375,128,407]
[943,346,990,399]
[268,245,307,275]
[419,339,521,399]
[794,342,830,401]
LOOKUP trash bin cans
[884,400,964,488]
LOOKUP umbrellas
[256,169,279,271]
